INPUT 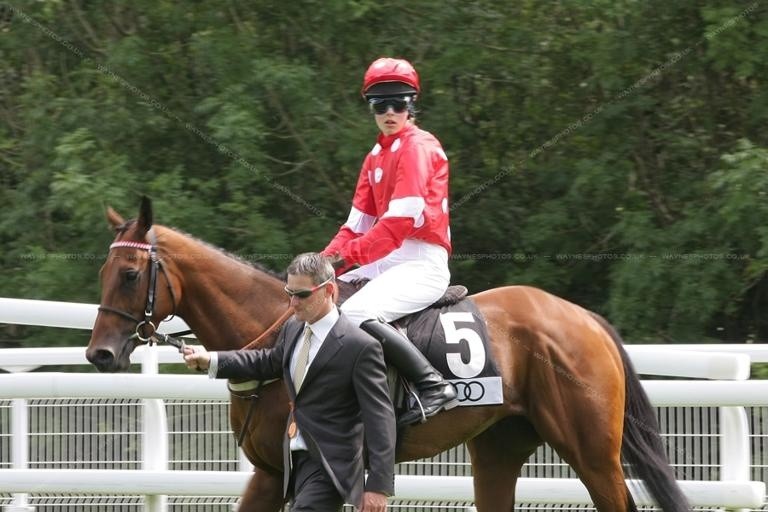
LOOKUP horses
[84,196,696,512]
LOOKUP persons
[319,58,460,427]
[181,253,398,512]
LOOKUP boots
[360,319,459,426]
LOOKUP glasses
[369,98,410,114]
[284,277,332,298]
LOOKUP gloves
[325,253,344,270]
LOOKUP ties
[293,327,312,395]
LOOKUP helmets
[361,58,420,101]
